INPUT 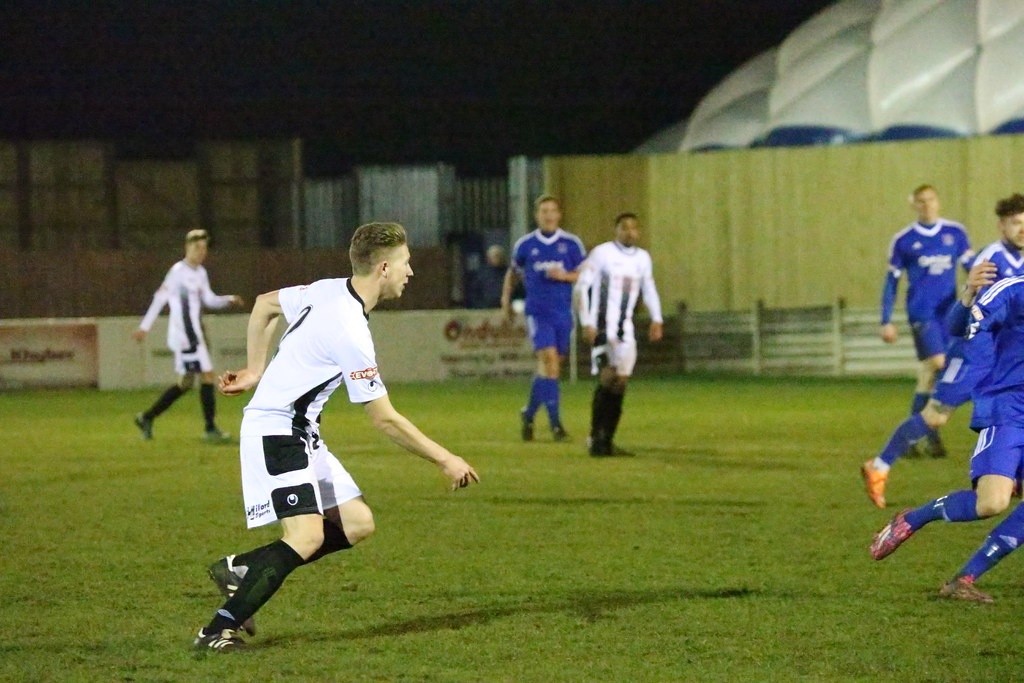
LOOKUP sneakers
[939,576,994,603]
[209,556,256,636]
[193,625,245,651]
[861,459,889,509]
[871,509,914,560]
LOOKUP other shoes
[521,408,534,440]
[137,415,152,439]
[203,430,228,442]
[903,445,921,457]
[928,441,945,456]
[554,428,569,440]
[587,438,623,455]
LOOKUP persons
[869,259,1024,603]
[500,194,587,443]
[134,229,243,440]
[860,194,1024,509]
[879,184,974,459]
[191,221,479,654]
[473,245,522,308]
[571,213,664,458]
